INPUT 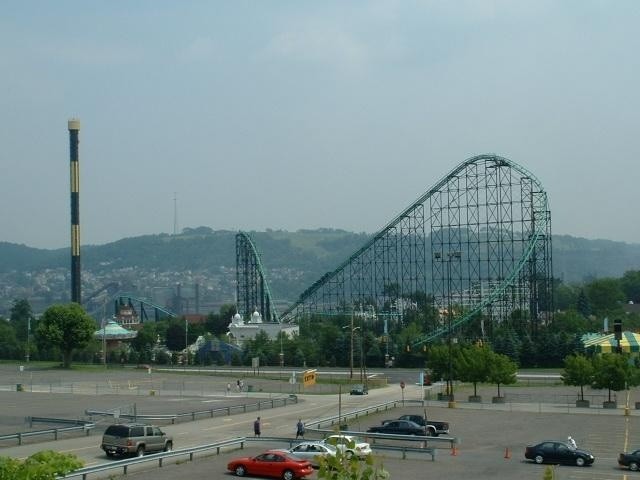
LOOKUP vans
[350,384,368,395]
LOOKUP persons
[253,416,262,438]
[226,382,232,393]
[566,436,578,450]
[296,419,306,440]
[238,378,244,392]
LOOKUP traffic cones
[450,444,459,456]
[503,446,512,458]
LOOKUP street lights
[433,250,463,409]
[342,298,361,377]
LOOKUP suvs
[100,423,172,461]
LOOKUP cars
[525,439,595,467]
[617,449,640,471]
[318,435,373,461]
[268,441,344,471]
[228,449,314,479]
[366,420,429,440]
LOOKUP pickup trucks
[382,414,450,438]
